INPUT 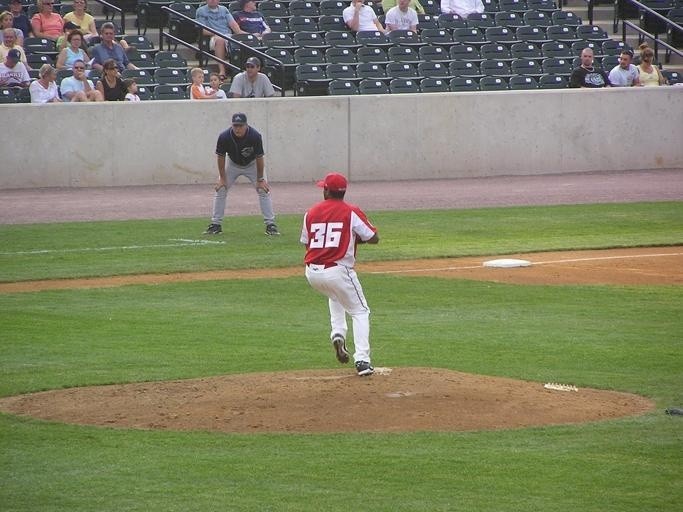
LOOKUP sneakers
[265,226,280,235]
[356,364,373,376]
[205,224,222,235]
[331,334,350,363]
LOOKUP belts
[307,261,338,268]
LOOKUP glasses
[75,66,85,70]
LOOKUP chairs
[0,0,683,107]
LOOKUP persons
[202,112,281,237]
[299,175,379,377]
[571,48,612,88]
[608,50,640,87]
[635,41,664,87]
[196,0,240,80]
[230,57,275,97]
[343,0,486,35]
[190,68,206,98]
[232,0,272,41]
[0,0,141,101]
[200,72,228,98]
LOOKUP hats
[317,173,347,192]
[7,50,21,62]
[63,22,79,30]
[245,58,260,68]
[232,114,247,125]
